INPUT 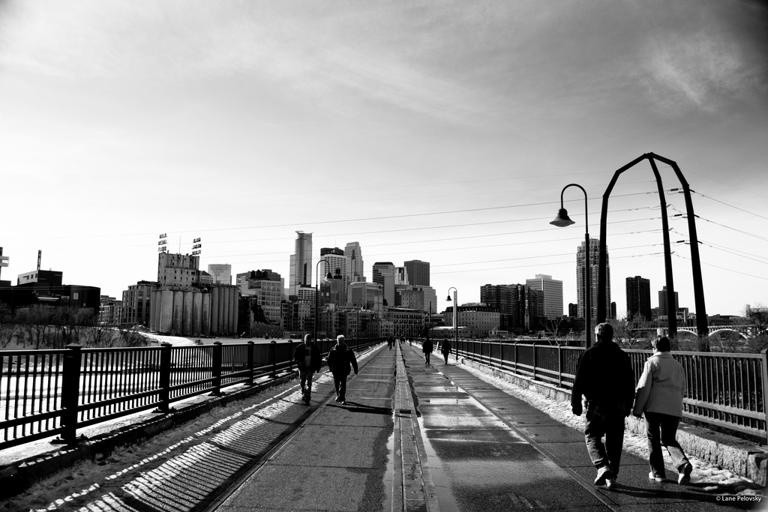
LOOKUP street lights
[550,184,591,349]
[314,259,341,344]
[423,300,434,343]
[354,302,367,352]
[446,286,459,360]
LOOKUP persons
[326,334,359,405]
[381,334,413,352]
[570,322,636,488]
[631,337,693,485]
[294,333,322,405]
[421,337,433,365]
[440,338,452,365]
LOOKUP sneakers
[605,477,616,489]
[593,465,612,485]
[648,471,666,483]
[677,462,692,485]
[301,393,346,405]
